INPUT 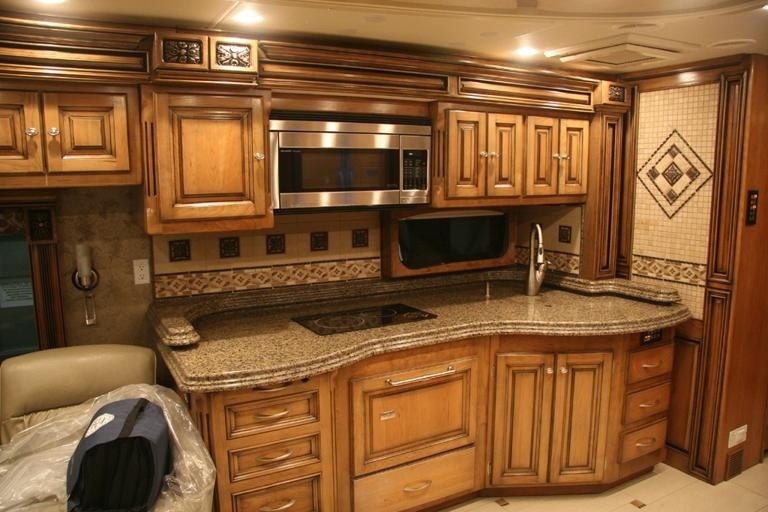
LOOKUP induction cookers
[288,302,438,337]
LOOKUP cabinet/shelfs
[616,341,675,464]
[168,371,335,512]
[151,92,267,221]
[348,352,479,512]
[489,351,613,486]
[1,89,130,175]
[445,108,524,199]
[525,114,589,196]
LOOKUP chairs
[0,343,217,511]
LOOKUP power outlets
[132,258,150,285]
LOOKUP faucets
[525,223,552,296]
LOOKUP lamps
[71,237,100,291]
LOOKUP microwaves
[269,116,434,212]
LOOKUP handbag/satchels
[66,397,172,512]
